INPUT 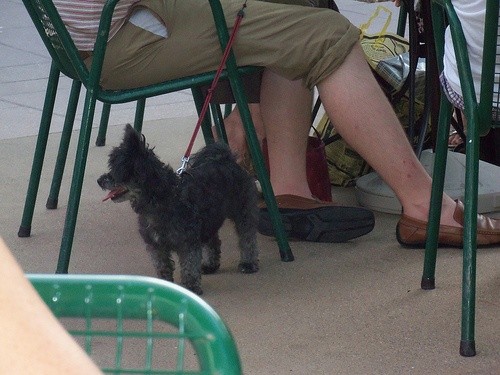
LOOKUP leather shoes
[256,193,374,242]
[397,199,500,248]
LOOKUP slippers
[448,124,489,148]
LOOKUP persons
[30,0,500,248]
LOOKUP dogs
[96,123,260,296]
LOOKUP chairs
[17,0,500,375]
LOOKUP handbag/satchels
[313,5,455,186]
[262,124,332,203]
